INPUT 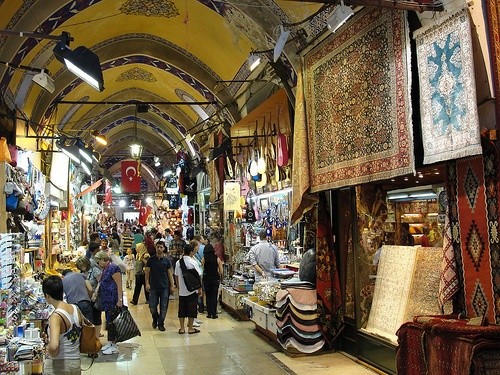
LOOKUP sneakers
[101,342,111,350]
[103,344,118,355]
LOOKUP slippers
[189,328,201,334]
[179,329,185,334]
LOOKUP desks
[221,287,252,321]
[241,298,278,345]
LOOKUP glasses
[96,261,100,264]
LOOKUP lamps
[153,81,268,178]
[127,110,143,159]
[54,129,107,175]
[0,61,56,95]
[0,27,105,92]
[273,3,333,62]
[247,27,308,72]
[327,0,354,34]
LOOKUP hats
[94,250,110,259]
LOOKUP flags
[120,159,141,193]
[133,199,141,209]
[139,207,151,226]
[105,179,113,205]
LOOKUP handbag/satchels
[7,143,19,167]
[5,164,50,221]
[0,136,11,163]
[108,305,142,343]
[182,269,202,292]
[134,260,145,274]
[77,305,103,354]
[16,147,28,173]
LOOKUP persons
[61,217,225,359]
[42,274,82,375]
[174,244,202,334]
[248,229,280,283]
[90,250,124,355]
[144,241,176,331]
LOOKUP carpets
[359,245,452,346]
[264,348,388,375]
[413,3,482,164]
[303,4,417,194]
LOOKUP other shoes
[216,310,222,314]
[130,299,137,304]
[145,300,149,304]
[198,309,204,313]
[159,322,167,331]
[188,318,203,327]
[152,318,159,328]
[206,314,218,319]
[126,284,132,289]
